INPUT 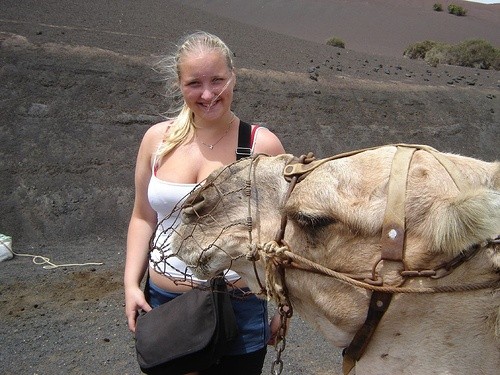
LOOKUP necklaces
[197,114,235,150]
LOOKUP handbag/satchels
[134,268,240,375]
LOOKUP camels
[171,143,500,375]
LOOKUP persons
[124,28,289,375]
